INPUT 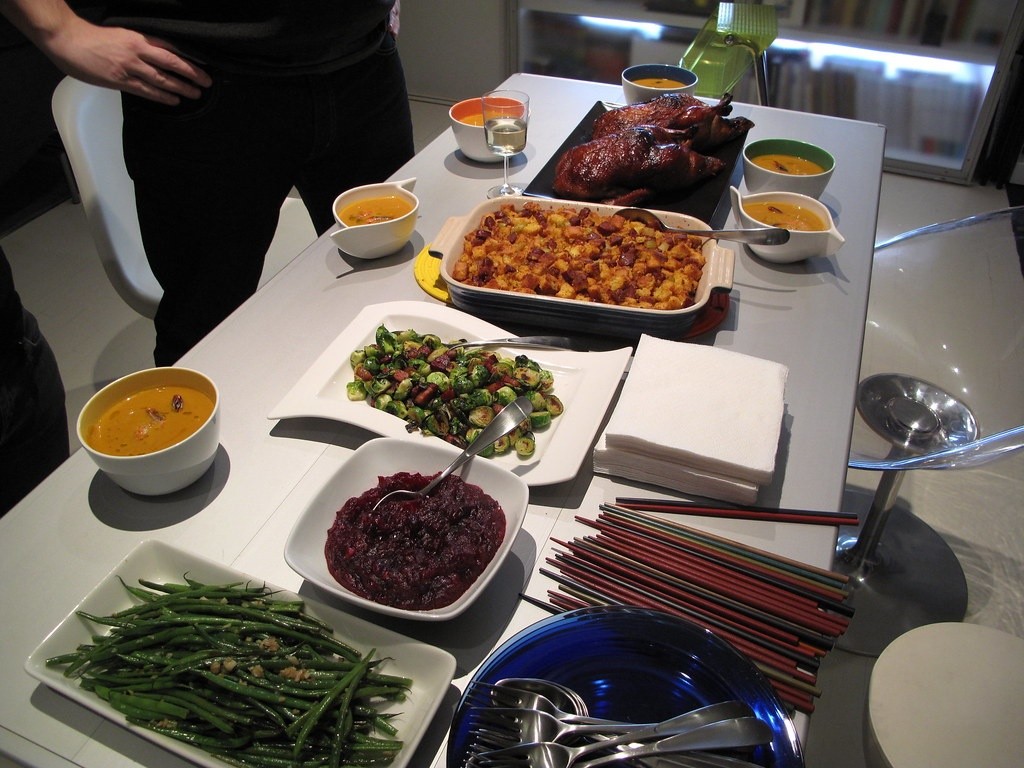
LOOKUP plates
[266,300,633,488]
[24,538,457,768]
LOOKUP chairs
[51,75,318,321]
[828,206,1024,656]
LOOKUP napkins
[593,334,789,506]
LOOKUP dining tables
[0,72,887,768]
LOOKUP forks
[453,680,775,768]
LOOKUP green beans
[44,570,415,768]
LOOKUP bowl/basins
[448,97,527,164]
[329,176,420,259]
[729,185,845,265]
[429,196,736,338]
[521,100,752,225]
[621,63,699,105]
[75,366,223,496]
[280,437,531,623]
[446,603,805,768]
[742,138,836,199]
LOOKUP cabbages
[343,325,564,456]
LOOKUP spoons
[368,395,535,517]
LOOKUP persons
[0,1,415,367]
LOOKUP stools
[863,622,1024,768]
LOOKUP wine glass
[480,90,530,200]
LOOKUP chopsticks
[517,497,862,715]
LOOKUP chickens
[592,92,755,150]
[552,123,727,207]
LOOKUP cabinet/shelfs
[517,0,1024,187]
